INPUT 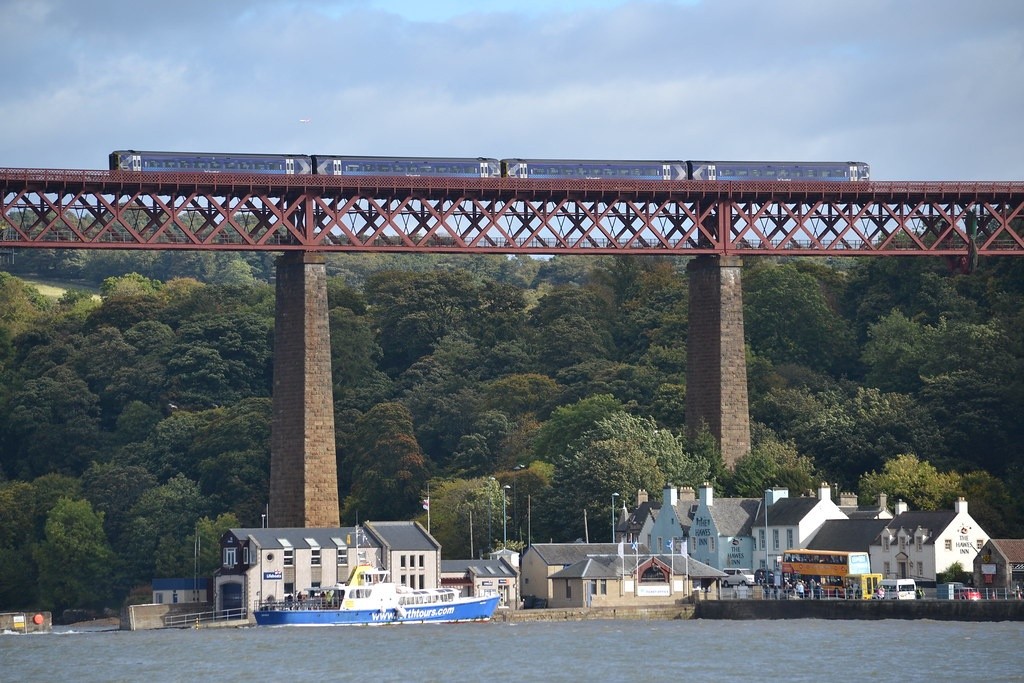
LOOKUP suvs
[754,568,775,585]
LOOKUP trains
[108,148,871,184]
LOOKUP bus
[781,548,870,598]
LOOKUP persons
[765,584,770,599]
[773,583,778,598]
[877,585,885,600]
[297,591,304,610]
[287,591,293,610]
[787,578,823,599]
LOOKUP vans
[872,578,917,600]
[721,568,756,587]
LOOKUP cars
[954,587,984,600]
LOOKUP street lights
[488,476,495,553]
[611,492,620,544]
[765,488,772,587]
[513,466,522,541]
[503,485,511,548]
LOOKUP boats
[252,510,502,627]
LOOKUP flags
[632,538,637,551]
[422,497,427,508]
[667,538,672,549]
